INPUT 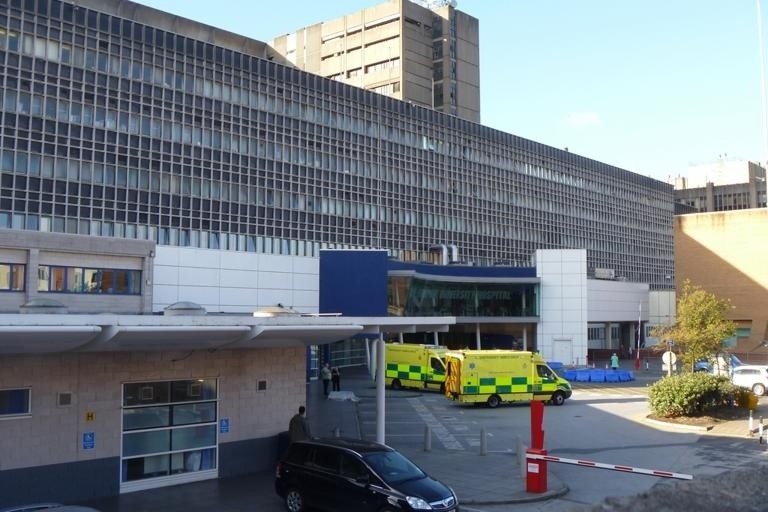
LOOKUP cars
[692,354,746,375]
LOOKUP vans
[275,435,460,511]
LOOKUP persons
[331,365,341,391]
[287,406,313,442]
[620,343,626,360]
[609,352,620,370]
[320,362,333,395]
[626,344,633,360]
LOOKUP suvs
[1,502,101,511]
[718,364,768,395]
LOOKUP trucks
[374,342,452,395]
[444,346,573,406]
